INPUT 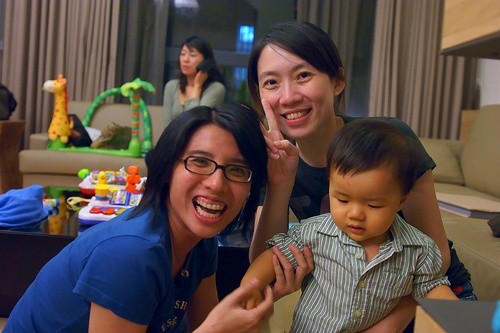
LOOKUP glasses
[177,154,253,183]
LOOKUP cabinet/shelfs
[441,0,500,61]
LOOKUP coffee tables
[0,192,251,333]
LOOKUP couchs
[19,99,167,190]
[419,103,500,300]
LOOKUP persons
[249,21,477,333]
[239,116,460,333]
[164,37,226,129]
[0,102,314,333]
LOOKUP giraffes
[41,73,75,143]
